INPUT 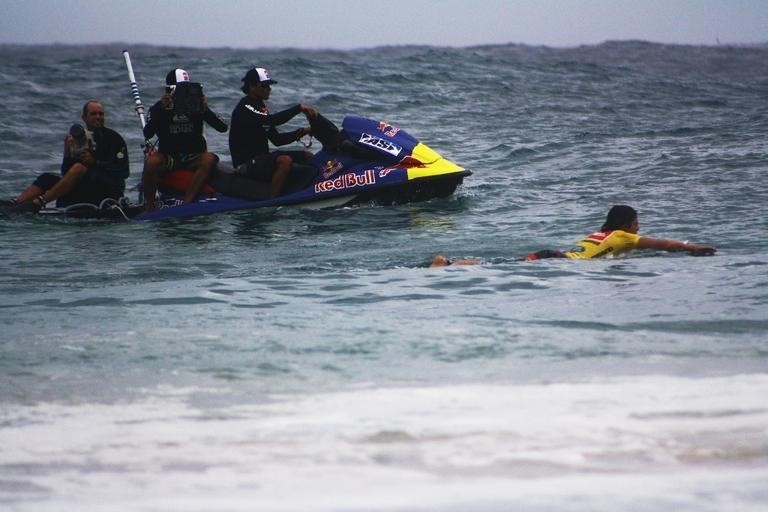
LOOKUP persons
[1,100,132,214]
[144,69,228,211]
[426,206,717,268]
[230,68,317,200]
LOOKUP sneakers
[0,198,39,219]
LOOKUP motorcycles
[0,100,475,226]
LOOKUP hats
[166,68,190,83]
[241,67,278,85]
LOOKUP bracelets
[680,239,689,249]
